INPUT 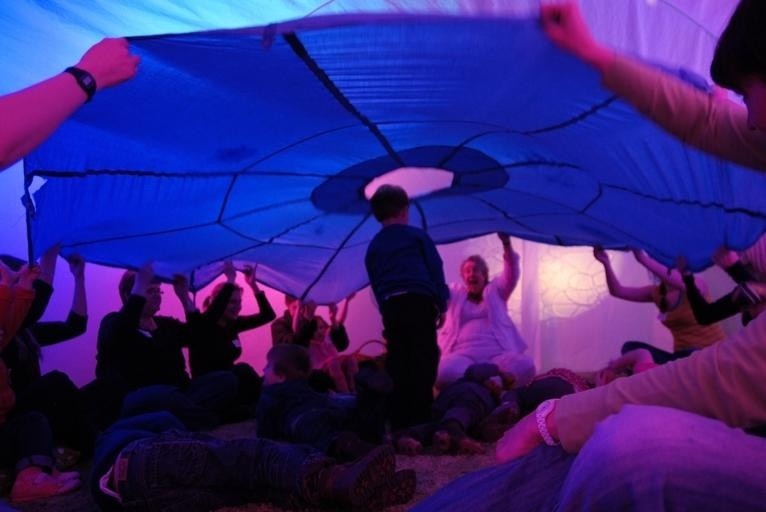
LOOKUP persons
[411,246,765,510]
[435,234,535,388]
[363,183,452,433]
[405,0,766,510]
[0,34,144,170]
[538,0,765,180]
[0,240,416,511]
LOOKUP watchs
[66,63,100,107]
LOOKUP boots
[306,443,416,512]
